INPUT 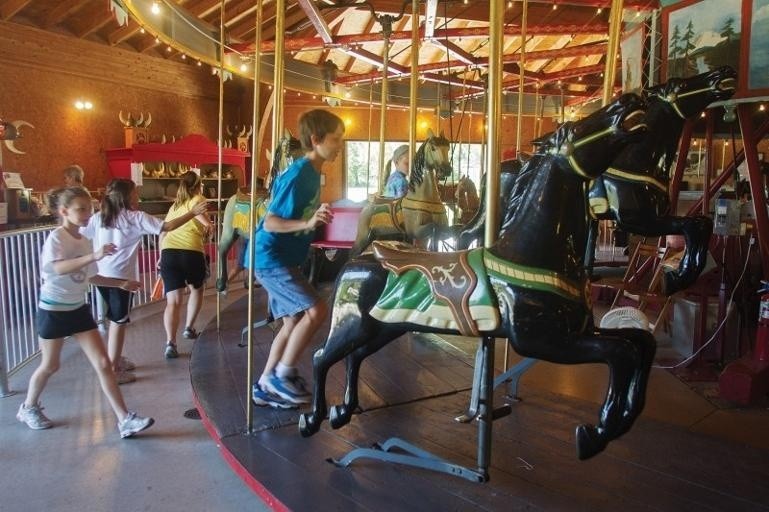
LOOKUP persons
[14,184,155,440]
[156,170,215,359]
[378,145,417,202]
[61,165,94,218]
[243,107,348,409]
[80,177,209,385]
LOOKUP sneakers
[112,367,137,386]
[263,368,314,403]
[183,326,200,338]
[117,411,155,438]
[253,383,299,410]
[164,345,179,357]
[15,402,54,431]
[114,358,135,369]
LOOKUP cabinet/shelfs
[105,147,251,218]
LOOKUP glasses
[62,174,74,180]
